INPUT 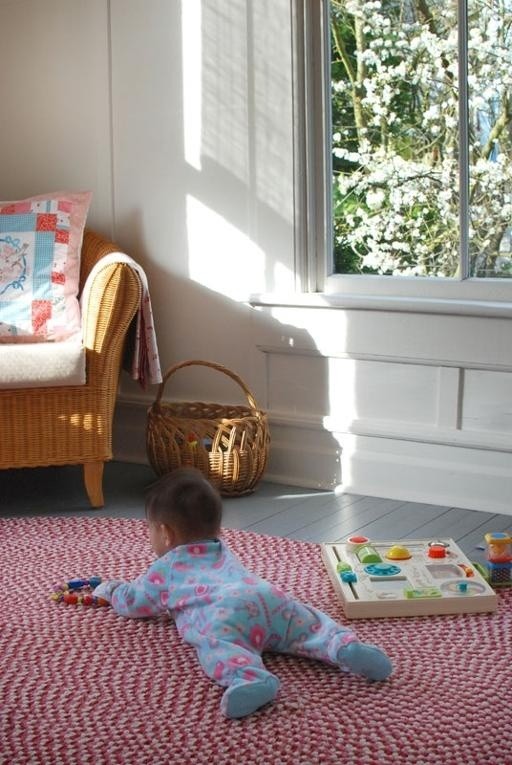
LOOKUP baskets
[139,354,277,502]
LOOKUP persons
[94,463,393,719]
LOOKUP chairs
[0,229,143,508]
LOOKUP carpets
[0,514,512,764]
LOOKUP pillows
[0,189,94,341]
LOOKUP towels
[80,253,164,386]
